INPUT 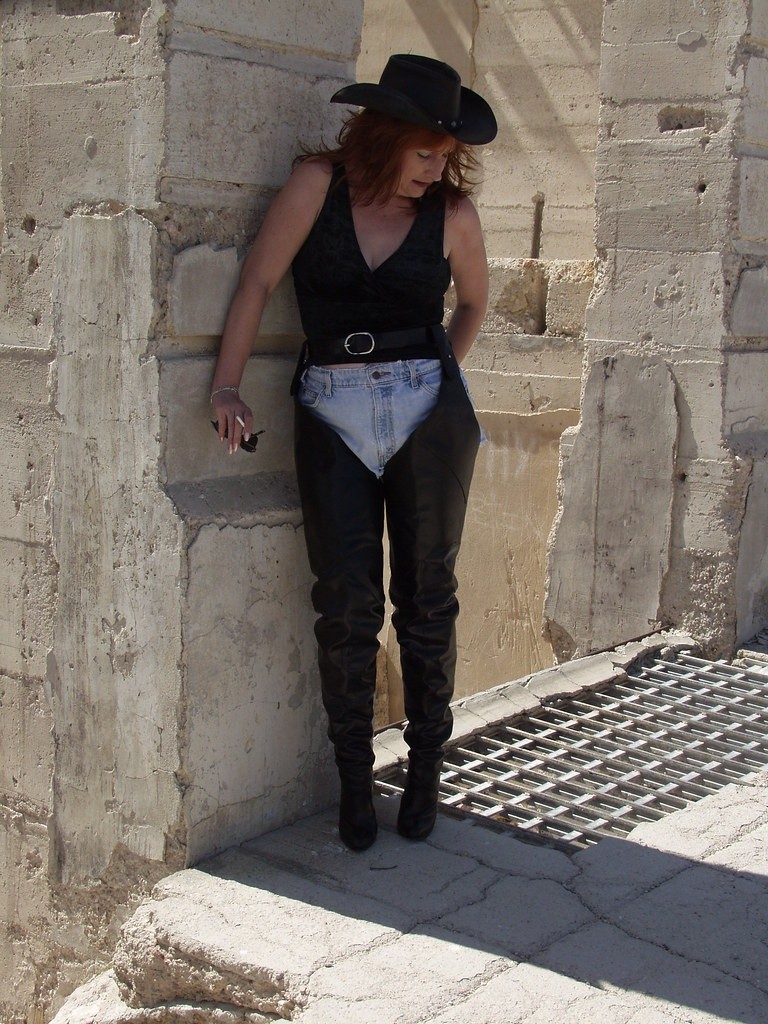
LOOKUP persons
[213,54,499,855]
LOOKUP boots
[293,364,385,850]
[385,356,481,840]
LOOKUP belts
[308,324,446,356]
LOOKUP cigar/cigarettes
[236,415,245,428]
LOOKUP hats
[329,54,498,145]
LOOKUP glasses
[210,419,266,453]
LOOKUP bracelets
[211,386,239,402]
